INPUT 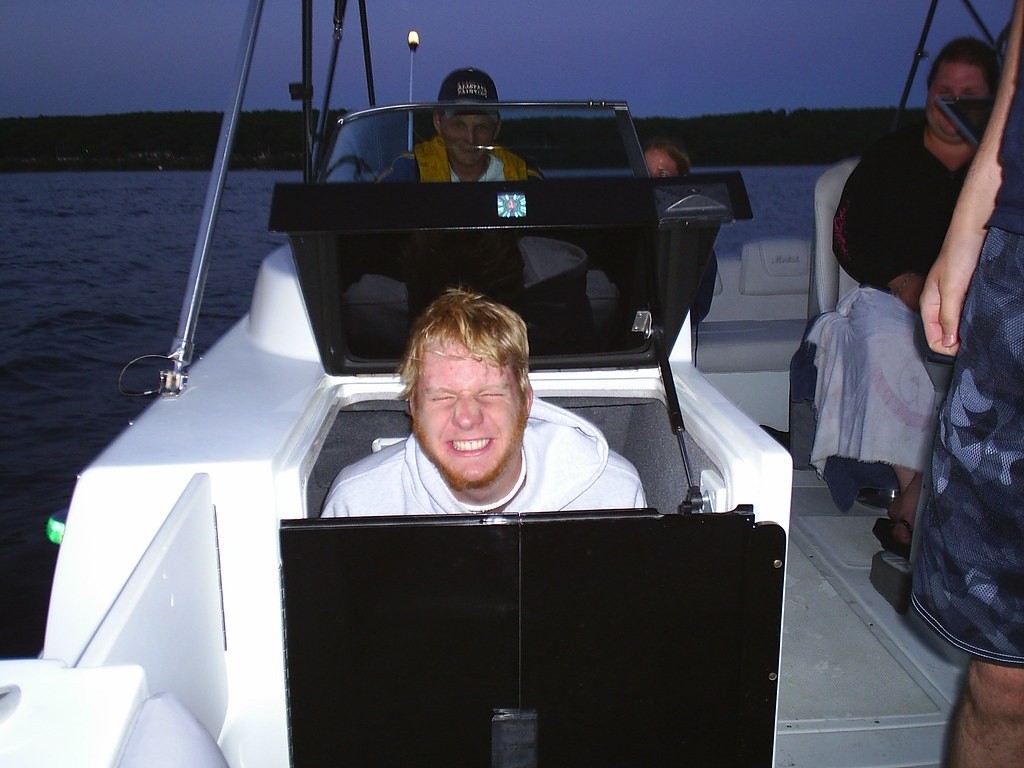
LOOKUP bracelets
[894,274,914,297]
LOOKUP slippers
[872,518,913,560]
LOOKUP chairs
[305,397,724,521]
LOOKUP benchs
[690,236,814,374]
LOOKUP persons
[830,36,997,560]
[910,0,1024,767]
[640,138,690,179]
[373,67,544,182]
[316,287,648,519]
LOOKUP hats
[437,67,498,114]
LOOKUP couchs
[814,158,867,315]
